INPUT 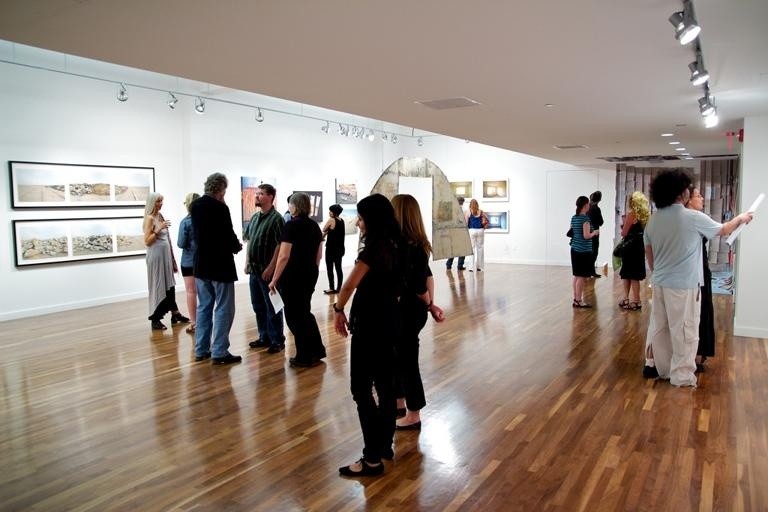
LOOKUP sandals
[186,324,195,333]
[574,300,592,307]
[624,302,640,310]
[619,299,628,306]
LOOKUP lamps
[113,81,428,146]
[665,0,720,131]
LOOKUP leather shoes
[324,290,334,293]
[290,358,314,366]
[396,420,421,430]
[397,408,406,417]
[213,354,241,364]
[250,336,270,347]
[268,344,285,352]
[644,366,658,378]
[195,352,211,361]
[339,458,384,476]
[382,448,394,459]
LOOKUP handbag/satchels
[481,211,487,225]
[613,239,633,257]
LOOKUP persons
[176,192,202,333]
[684,186,714,373]
[241,185,287,355]
[643,306,660,380]
[143,192,191,329]
[586,191,604,277]
[445,196,465,268]
[283,194,293,224]
[389,195,446,431]
[190,173,245,364]
[330,192,407,477]
[612,191,651,310]
[322,202,346,294]
[643,169,755,386]
[464,199,490,270]
[568,197,597,307]
[269,193,328,368]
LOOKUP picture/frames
[12,215,147,267]
[483,211,509,234]
[481,180,510,202]
[7,160,155,209]
[292,190,323,223]
[449,180,474,203]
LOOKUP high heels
[152,320,167,330]
[171,313,189,323]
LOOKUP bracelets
[333,303,342,317]
[153,231,159,236]
[426,300,435,310]
